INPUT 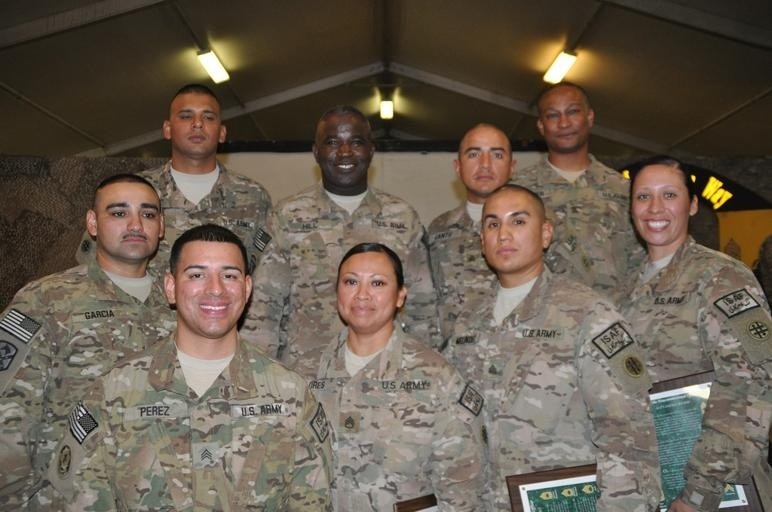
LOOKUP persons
[310,240,494,512]
[606,153,771,512]
[438,181,663,512]
[44,223,334,512]
[237,106,443,386]
[506,84,644,298]
[420,120,594,344]
[74,83,275,277]
[1,172,180,511]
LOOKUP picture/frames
[392,493,440,512]
[505,462,662,512]
[648,369,765,512]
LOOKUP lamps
[197,49,231,84]
[543,50,578,85]
[380,100,394,119]
